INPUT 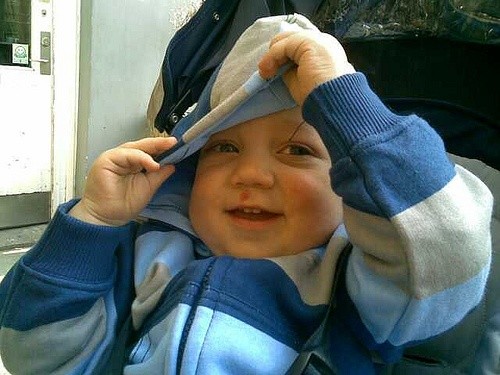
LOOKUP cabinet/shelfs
[0,0,31,68]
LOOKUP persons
[1,12,497,374]
